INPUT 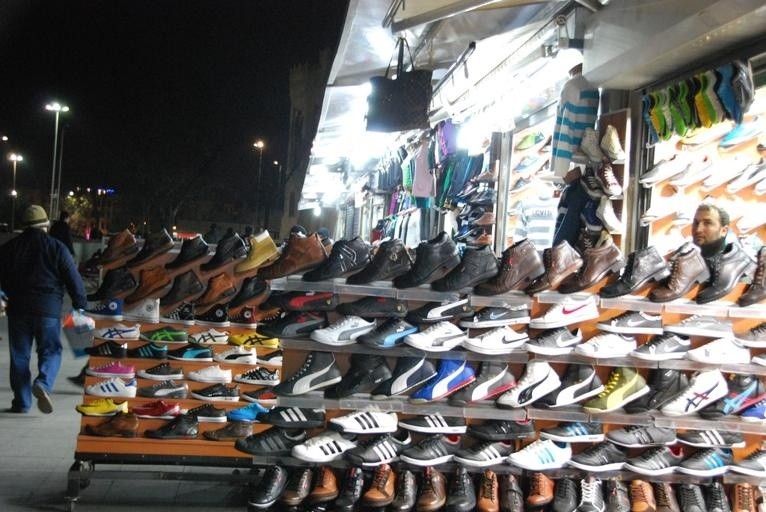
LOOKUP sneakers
[580,58,765,235]
[76,227,766,512]
[453,132,552,245]
[31,383,53,414]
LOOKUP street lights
[8,151,22,231]
[43,100,69,215]
[272,158,282,192]
[250,139,264,228]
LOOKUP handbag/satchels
[366,69,433,133]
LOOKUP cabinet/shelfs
[251,244,765,512]
[504,122,553,244]
[75,235,283,480]
[643,86,766,249]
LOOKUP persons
[689,203,730,259]
[549,167,589,250]
[203,222,332,249]
[550,63,599,166]
[48,208,77,257]
[0,202,86,417]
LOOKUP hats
[22,205,51,228]
